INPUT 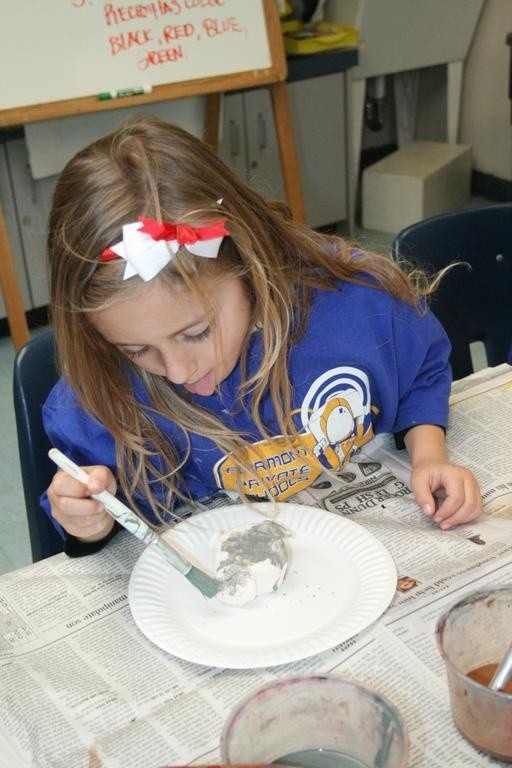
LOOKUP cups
[432,584,511,763]
[220,673,411,768]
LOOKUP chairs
[11,328,78,564]
[393,205,512,381]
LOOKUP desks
[0,361,512,768]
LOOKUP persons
[35,119,485,559]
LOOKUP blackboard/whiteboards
[0,0,289,129]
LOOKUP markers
[98,86,152,101]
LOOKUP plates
[122,498,404,675]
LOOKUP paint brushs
[48,447,223,598]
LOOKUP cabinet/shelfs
[0,139,62,322]
[217,70,348,229]
